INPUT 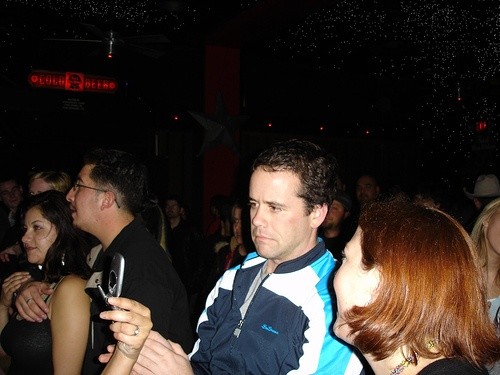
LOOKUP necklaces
[388,339,442,375]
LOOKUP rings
[132,324,140,336]
[25,296,32,304]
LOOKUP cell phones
[97,252,126,310]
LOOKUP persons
[65,153,197,375]
[99,296,154,375]
[0,189,93,375]
[1,167,29,230]
[160,189,254,290]
[331,192,500,375]
[0,171,73,261]
[98,139,367,375]
[473,198,500,375]
[316,197,353,261]
[324,153,500,201]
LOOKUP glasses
[166,205,179,210]
[73,182,121,209]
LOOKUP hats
[463,174,499,198]
